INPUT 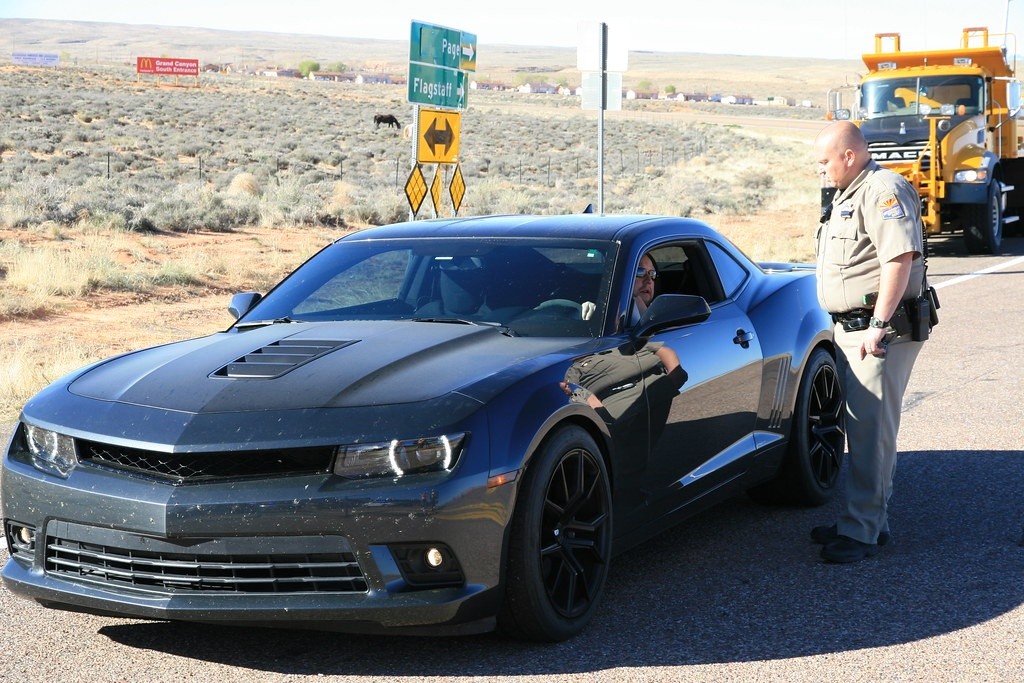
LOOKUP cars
[0,215,848,642]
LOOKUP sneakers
[821,535,880,562]
[810,524,890,545]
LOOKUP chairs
[413,257,494,316]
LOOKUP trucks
[820,29,1024,255]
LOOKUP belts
[597,367,658,402]
[831,308,873,325]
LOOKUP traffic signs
[409,21,478,108]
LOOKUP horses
[374,114,402,129]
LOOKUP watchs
[869,317,890,327]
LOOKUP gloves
[581,301,596,320]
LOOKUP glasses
[636,267,657,281]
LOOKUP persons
[561,339,688,476]
[809,121,928,563]
[584,251,658,327]
